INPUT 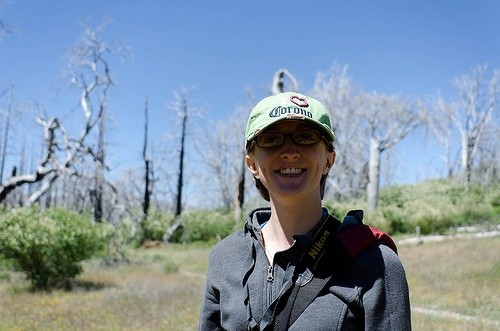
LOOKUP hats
[245,91,336,150]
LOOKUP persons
[198,92,412,331]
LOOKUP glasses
[248,132,332,152]
